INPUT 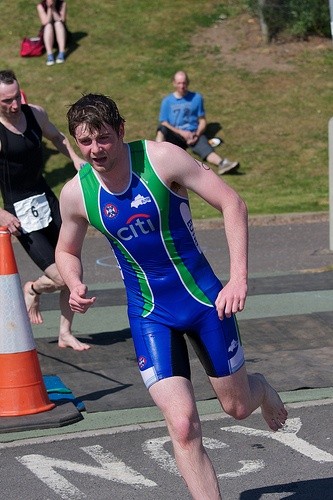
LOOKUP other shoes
[46,60,55,66]
[56,56,65,64]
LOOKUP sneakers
[218,158,239,175]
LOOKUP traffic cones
[0,225,55,420]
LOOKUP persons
[0,69,89,352]
[154,70,240,175]
[37,0,71,66]
[54,91,289,500]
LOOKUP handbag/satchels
[20,36,45,57]
